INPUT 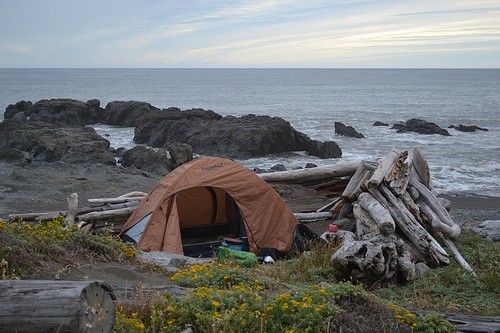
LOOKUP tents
[115,157,316,266]
